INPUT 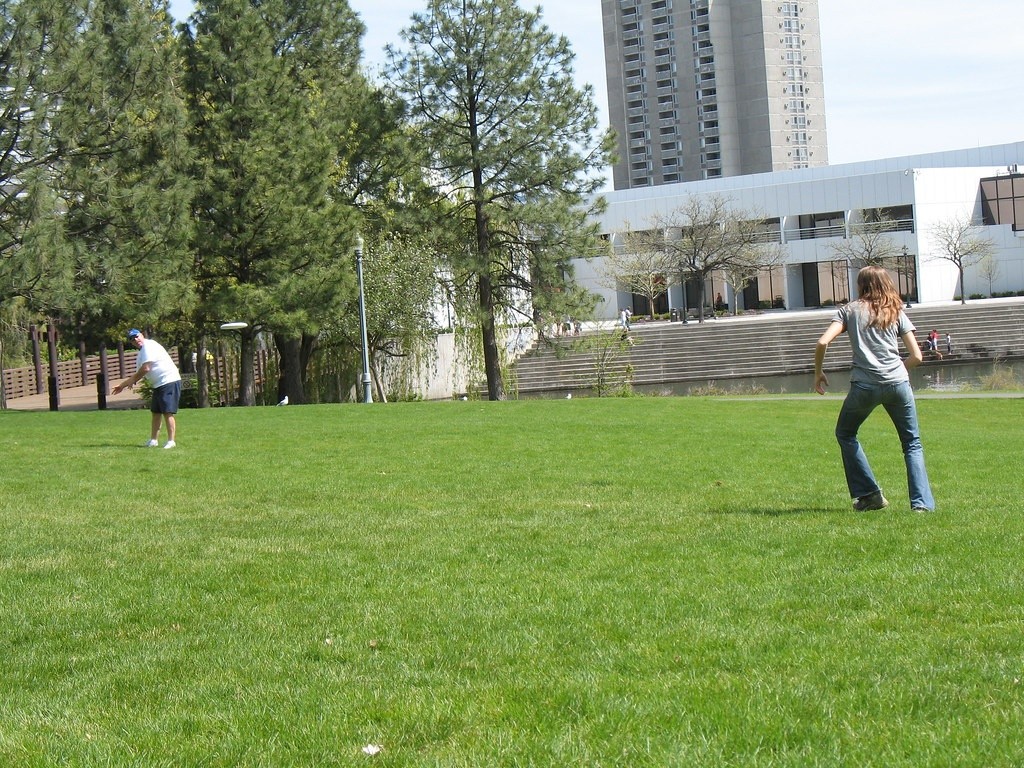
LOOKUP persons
[926,330,952,355]
[814,266,935,513]
[112,329,182,449]
[716,292,722,311]
[539,306,641,348]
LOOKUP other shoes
[164,441,176,449]
[147,440,158,447]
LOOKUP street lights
[355,232,373,404]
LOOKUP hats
[128,329,138,340]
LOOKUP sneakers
[915,508,925,513]
[853,493,888,511]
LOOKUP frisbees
[220,322,248,330]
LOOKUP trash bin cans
[671,308,677,322]
[680,307,688,321]
